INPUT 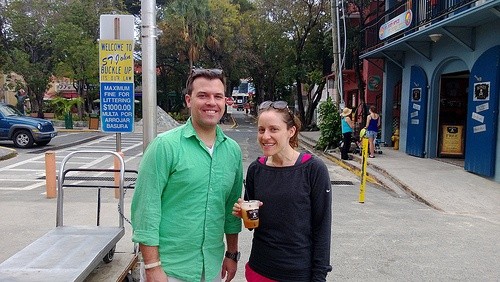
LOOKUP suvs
[0,102,57,148]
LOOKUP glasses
[259,100,293,120]
[191,69,224,80]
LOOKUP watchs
[225,251,240,263]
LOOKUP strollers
[352,127,382,156]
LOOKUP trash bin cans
[89,113,99,130]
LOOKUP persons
[15,90,27,111]
[232,101,332,282]
[339,108,354,160]
[365,106,380,158]
[24,105,27,115]
[131,70,244,282]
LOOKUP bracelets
[145,261,161,269]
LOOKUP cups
[239,200,260,228]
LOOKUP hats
[340,108,352,116]
